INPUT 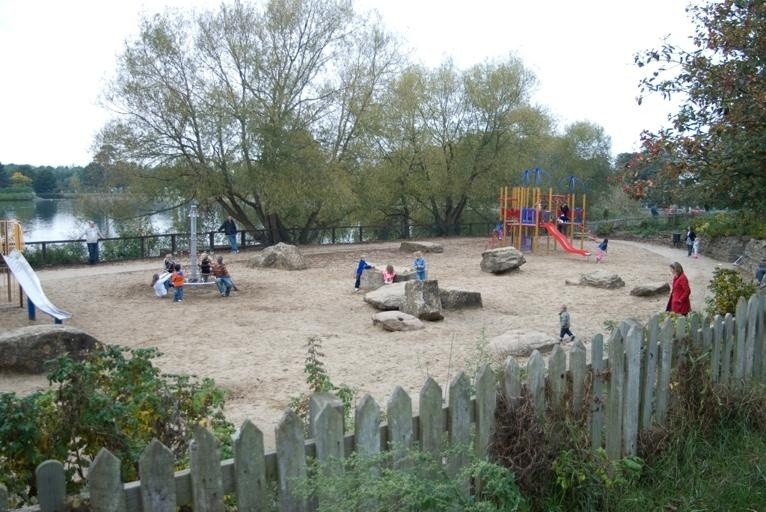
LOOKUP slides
[539,222,592,256]
[1,248,73,320]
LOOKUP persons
[413,250,427,280]
[595,238,609,264]
[495,217,503,241]
[383,264,396,284]
[534,198,544,225]
[75,220,106,265]
[651,204,659,216]
[665,262,692,318]
[218,215,239,255]
[151,250,240,305]
[558,201,570,223]
[355,254,375,291]
[685,226,698,257]
[558,304,575,341]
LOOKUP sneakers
[232,249,240,255]
[220,292,228,297]
[569,334,575,341]
